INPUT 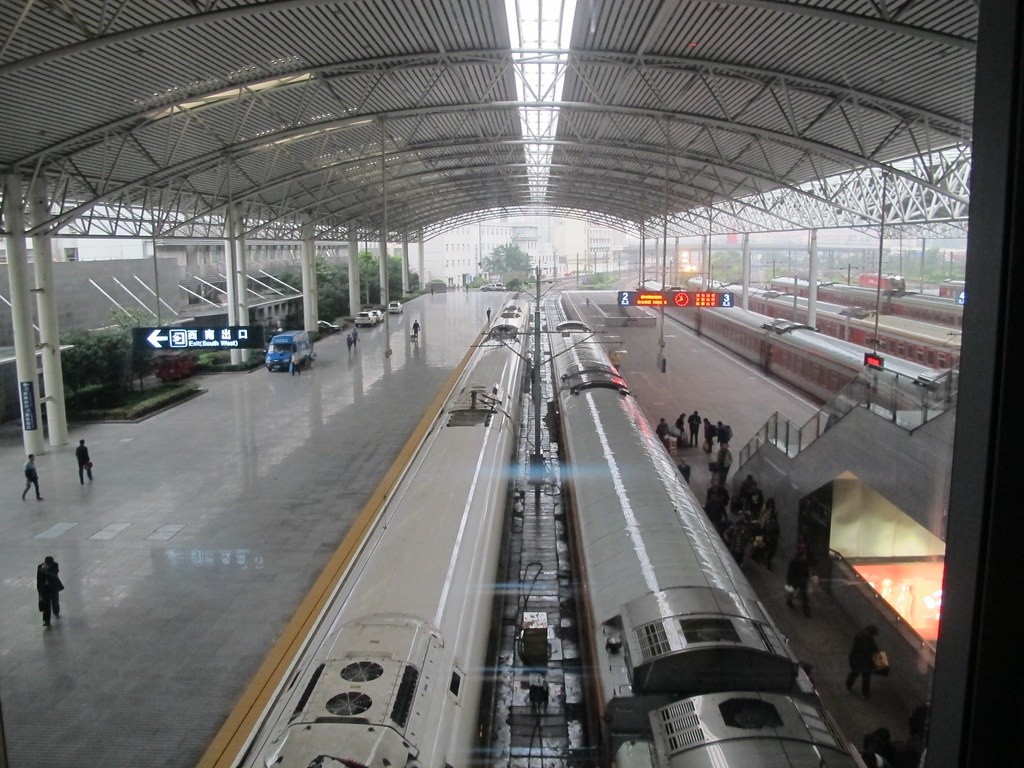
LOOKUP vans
[262,330,312,373]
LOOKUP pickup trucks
[480,283,506,292]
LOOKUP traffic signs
[132,327,265,349]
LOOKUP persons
[716,421,728,448]
[75,439,93,485]
[352,328,358,347]
[466,283,468,292]
[586,297,590,307]
[21,454,43,501]
[37,556,60,626]
[909,703,926,742]
[346,334,353,354]
[678,457,691,484]
[412,320,420,340]
[688,410,701,447]
[786,544,820,620]
[717,443,734,485]
[431,286,435,296]
[608,351,621,372]
[704,471,730,532]
[487,307,491,323]
[846,625,879,698]
[703,418,713,453]
[291,350,301,376]
[719,474,780,592]
[675,413,686,436]
[861,727,905,768]
[656,418,670,443]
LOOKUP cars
[318,321,340,335]
[354,312,378,328]
[387,301,402,314]
[370,309,384,323]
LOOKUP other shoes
[22,495,25,501]
[43,622,50,626]
[54,613,59,619]
[36,497,43,500]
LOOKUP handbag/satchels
[39,593,45,611]
[783,585,795,600]
[85,462,93,469]
[703,439,710,451]
[671,424,681,437]
[872,650,889,670]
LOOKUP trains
[548,320,867,767]
[637,273,965,431]
[230,298,532,768]
